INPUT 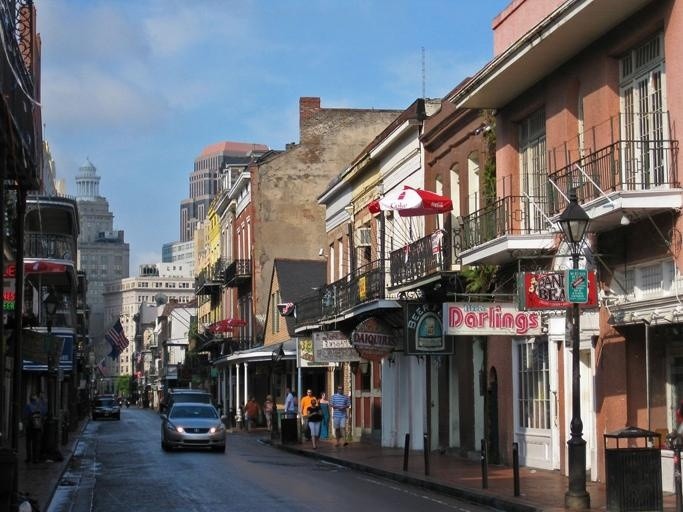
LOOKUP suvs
[160,387,224,421]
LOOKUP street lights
[269,341,285,442]
[553,186,598,511]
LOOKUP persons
[23,393,48,464]
[243,394,261,432]
[301,388,318,441]
[329,384,350,447]
[317,391,330,440]
[263,394,276,432]
[284,386,295,419]
[306,399,324,449]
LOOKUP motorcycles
[126,400,131,407]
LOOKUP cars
[93,394,121,420]
[161,402,228,452]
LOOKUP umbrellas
[207,319,246,328]
[207,324,232,334]
[368,184,454,241]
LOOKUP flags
[98,358,109,378]
[101,318,130,361]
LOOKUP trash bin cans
[0,449,17,512]
[604,426,663,512]
[280,411,298,443]
